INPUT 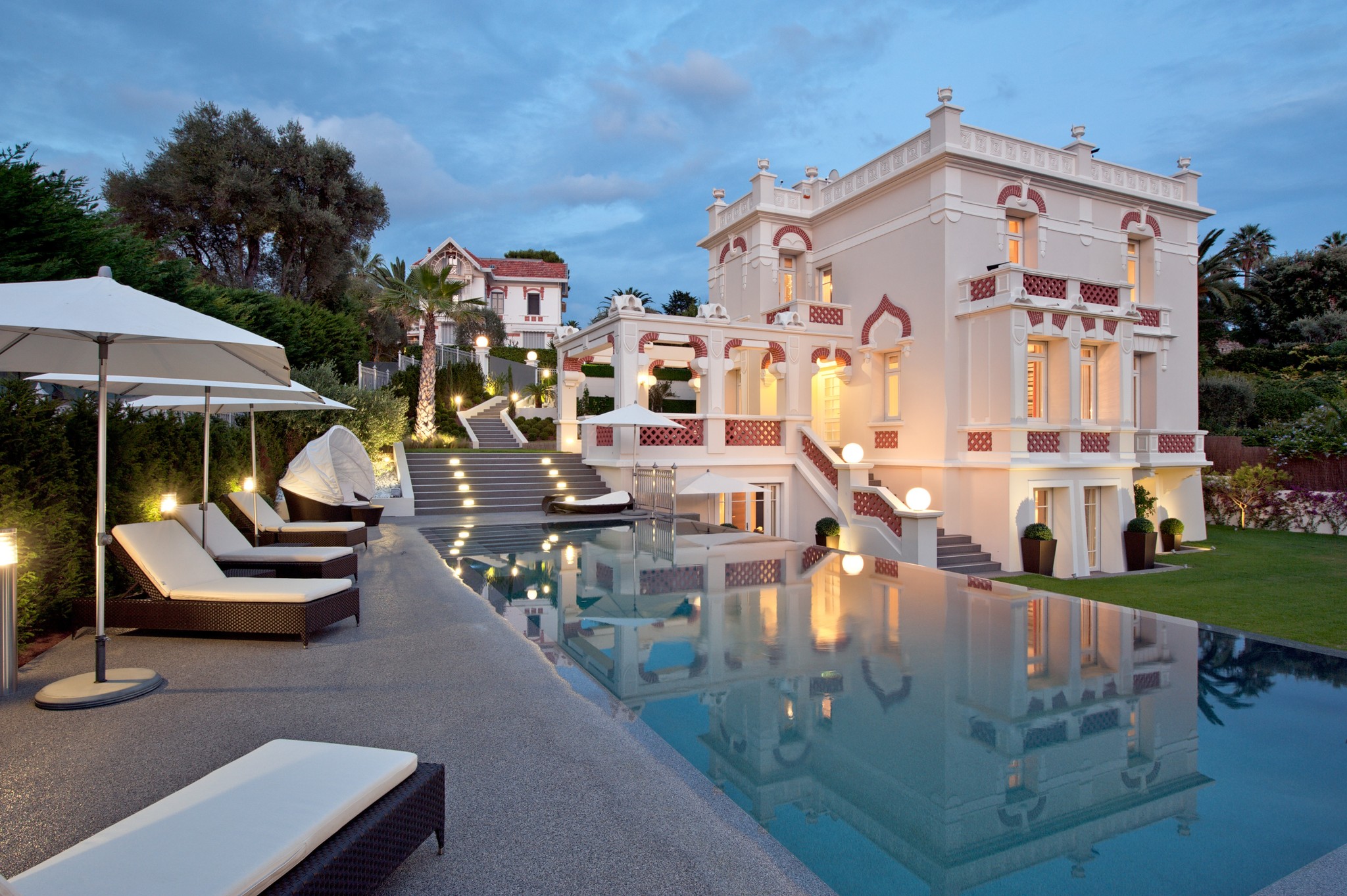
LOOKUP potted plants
[1123,519,1159,570]
[1161,517,1184,553]
[814,518,840,550]
[1020,523,1054,575]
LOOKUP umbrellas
[576,400,688,517]
[121,392,358,548]
[674,521,758,550]
[674,469,773,524]
[575,516,690,632]
[17,354,328,556]
[1,262,293,712]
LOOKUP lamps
[0,525,19,696]
[477,336,489,347]
[842,552,864,575]
[243,476,255,493]
[841,444,864,463]
[160,492,178,520]
[906,488,931,511]
[527,351,538,361]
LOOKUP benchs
[8,740,446,896]
[220,492,369,552]
[170,503,359,587]
[70,518,361,649]
